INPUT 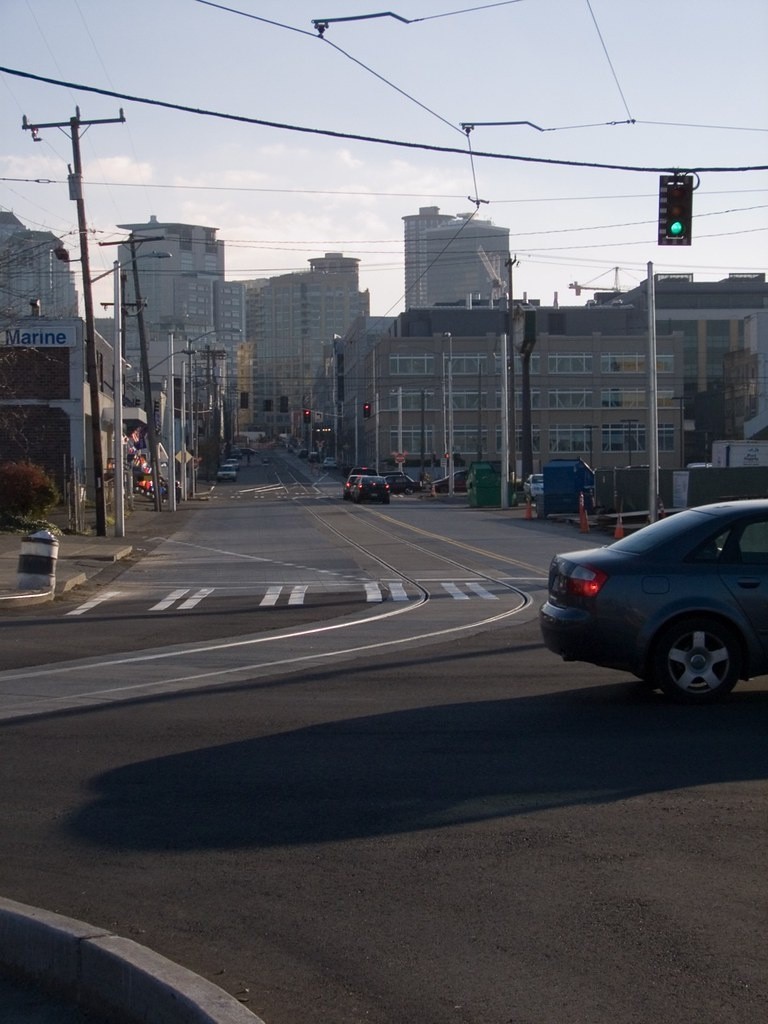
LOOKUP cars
[323,456,338,470]
[228,444,255,459]
[299,448,321,463]
[538,498,768,707]
[343,466,406,502]
[352,475,391,504]
[216,464,237,482]
[384,474,423,495]
[522,474,544,503]
[224,458,240,471]
[432,469,469,494]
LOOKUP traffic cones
[612,515,627,541]
[524,502,534,521]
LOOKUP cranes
[569,266,640,299]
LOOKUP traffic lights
[363,402,371,418]
[303,409,310,423]
[658,174,693,246]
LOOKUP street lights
[579,508,590,533]
[140,349,197,510]
[83,252,172,535]
[187,328,241,498]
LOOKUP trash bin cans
[465,460,517,507]
[542,457,595,515]
